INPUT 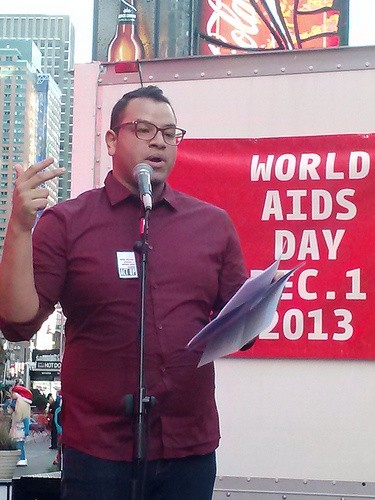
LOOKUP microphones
[132,162,153,212]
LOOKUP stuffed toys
[44,390,62,465]
[6,386,33,466]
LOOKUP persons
[0,85,276,500]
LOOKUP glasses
[111,121,187,147]
